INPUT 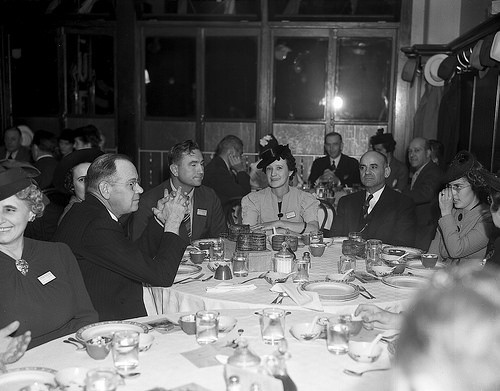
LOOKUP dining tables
[162,237,448,308]
[0,308,400,391]
[310,187,358,230]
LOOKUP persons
[201,136,251,226]
[1,126,112,194]
[250,135,304,194]
[1,160,98,353]
[309,132,365,189]
[470,166,500,277]
[395,138,444,218]
[240,145,321,244]
[426,152,500,266]
[369,127,411,193]
[55,155,192,325]
[1,322,32,373]
[128,139,230,249]
[327,151,418,249]
[58,148,106,231]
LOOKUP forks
[173,273,205,285]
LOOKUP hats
[469,31,500,78]
[442,151,474,181]
[424,54,454,86]
[437,57,459,86]
[256,144,286,169]
[34,130,57,152]
[61,128,74,144]
[0,158,41,201]
[401,56,419,87]
[65,148,106,169]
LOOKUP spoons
[278,296,285,304]
[344,368,389,377]
[178,275,213,284]
[363,333,383,355]
[271,292,283,304]
[359,292,370,300]
[359,286,375,299]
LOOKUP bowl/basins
[349,341,382,363]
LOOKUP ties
[363,195,372,216]
[183,213,191,240]
[8,151,13,160]
[331,161,336,168]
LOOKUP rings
[441,193,445,197]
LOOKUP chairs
[37,189,72,241]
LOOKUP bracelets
[300,222,308,235]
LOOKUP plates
[300,280,360,302]
[0,367,61,391]
[405,259,447,271]
[381,274,433,291]
[176,263,202,276]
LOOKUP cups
[302,178,354,202]
[53,306,365,391]
[181,224,439,284]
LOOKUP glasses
[112,177,141,188]
[446,184,471,191]
